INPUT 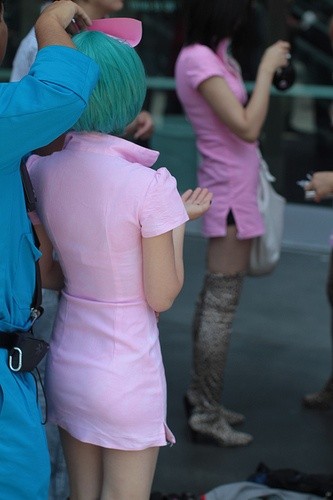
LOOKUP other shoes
[303,373,333,407]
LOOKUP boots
[182,275,246,427]
[187,270,254,447]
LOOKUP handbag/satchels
[250,151,287,279]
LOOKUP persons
[303,171,333,409]
[12,0,154,157]
[167,0,291,448]
[25,30,215,500]
[0,0,92,500]
